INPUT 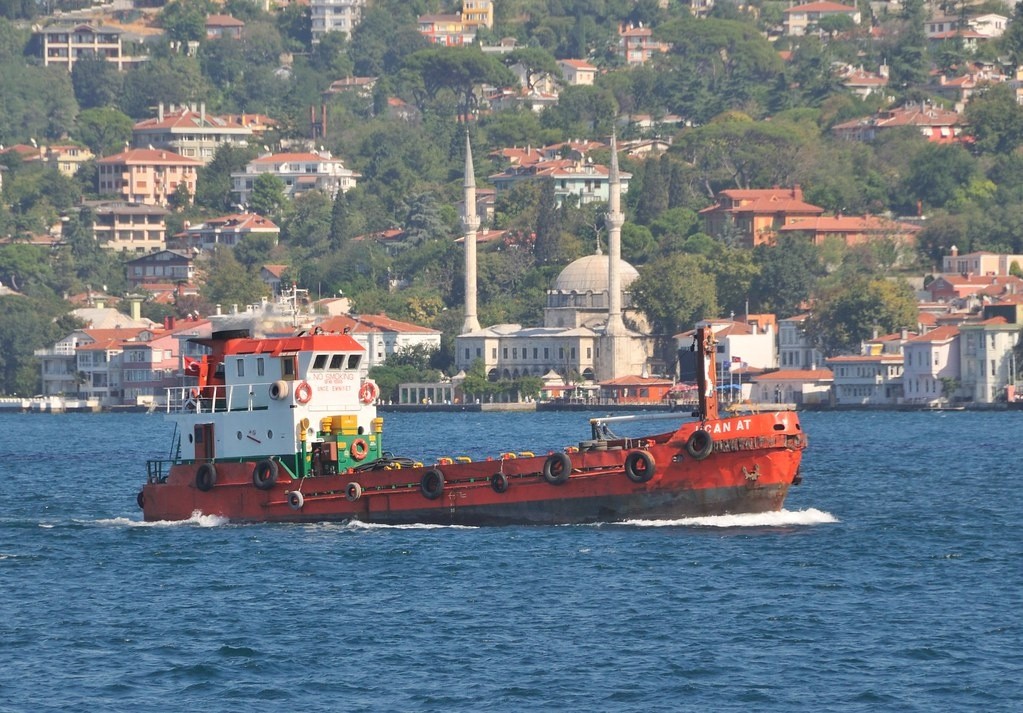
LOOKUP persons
[524,395,532,403]
[454,397,459,404]
[422,397,432,405]
[444,399,450,405]
[476,397,480,405]
[389,400,392,405]
[378,398,385,405]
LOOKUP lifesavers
[344,482,362,502]
[421,469,445,499]
[624,450,655,483]
[254,458,278,490]
[685,430,713,461]
[351,438,367,460]
[278,381,288,397]
[358,382,377,404]
[287,491,303,511]
[491,472,508,493]
[138,492,144,509]
[295,383,313,404]
[269,381,283,401]
[543,452,572,485]
[195,463,216,492]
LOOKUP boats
[136,328,808,527]
[922,396,965,411]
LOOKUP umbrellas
[669,382,698,391]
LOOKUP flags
[732,356,740,362]
[183,356,200,376]
[723,361,731,366]
